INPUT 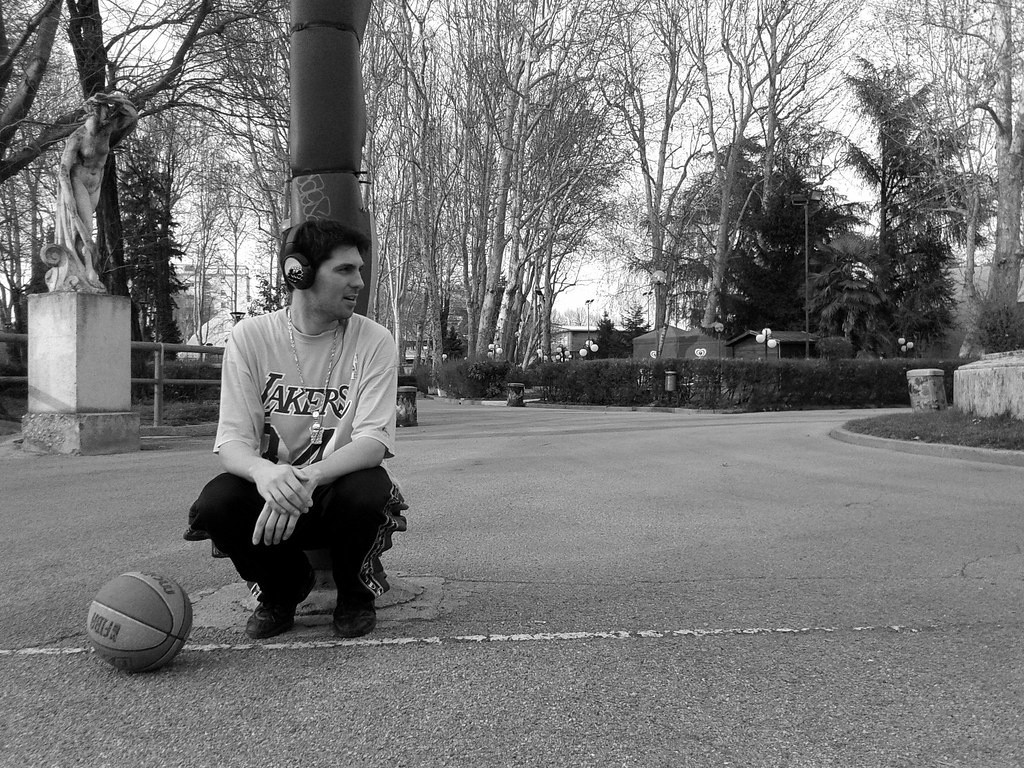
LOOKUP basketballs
[83,571,195,675]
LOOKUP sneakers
[333,586,376,638]
[246,565,317,639]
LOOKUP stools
[907,369,948,414]
[396,386,419,428]
[506,383,525,407]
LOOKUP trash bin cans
[663,369,678,393]
[506,381,525,408]
[396,374,419,428]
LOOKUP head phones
[282,221,313,290]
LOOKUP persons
[45,91,139,294]
[184,218,409,638]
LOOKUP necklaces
[288,306,340,444]
